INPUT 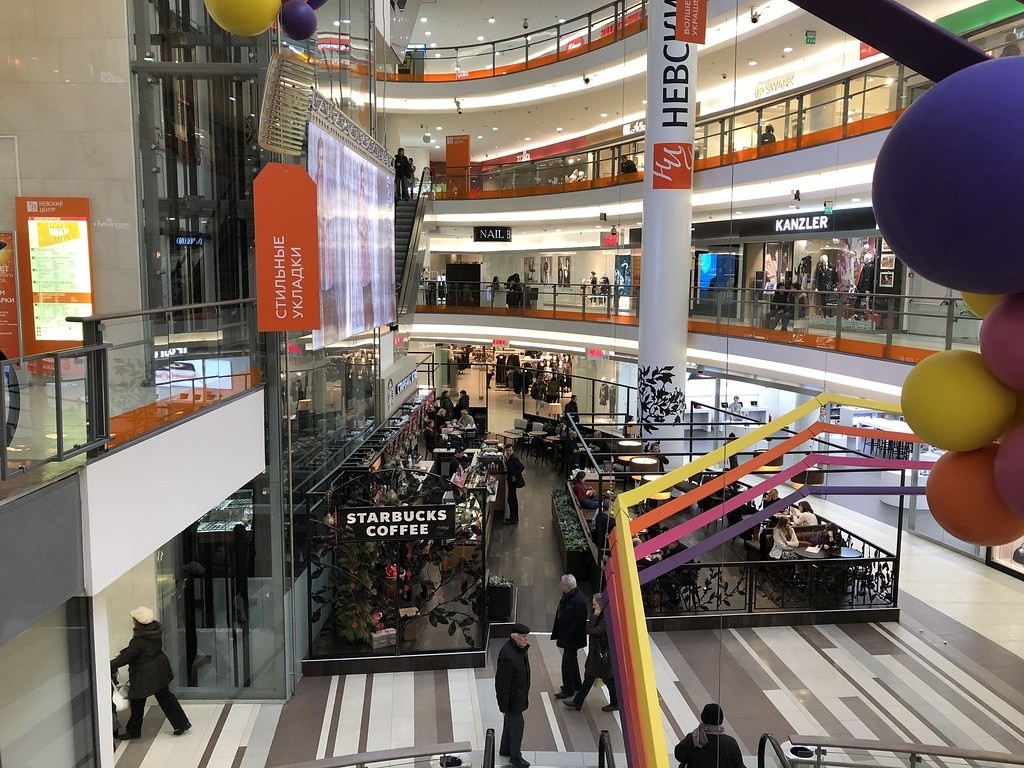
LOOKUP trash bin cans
[429,752,473,768]
[780,740,824,768]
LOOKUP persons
[723,433,739,468]
[391,148,416,200]
[617,155,637,183]
[496,352,506,385]
[550,575,619,712]
[578,271,609,305]
[423,390,526,526]
[815,245,874,321]
[486,358,494,389]
[1000,33,1021,57]
[599,377,616,421]
[563,395,845,585]
[762,124,776,157]
[526,257,570,287]
[111,608,192,740]
[315,137,375,344]
[492,273,523,307]
[674,703,748,768]
[544,353,571,392]
[528,349,558,414]
[225,524,251,624]
[765,282,806,332]
[495,624,531,768]
[615,258,631,294]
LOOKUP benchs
[745,525,829,561]
[573,490,597,520]
[759,291,810,333]
[762,525,835,575]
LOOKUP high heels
[564,700,581,711]
[602,704,619,711]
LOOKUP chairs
[424,423,479,461]
[698,476,873,609]
[636,560,702,612]
[504,419,565,476]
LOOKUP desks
[441,427,453,435]
[684,408,769,452]
[496,432,523,453]
[795,547,829,593]
[411,461,436,487]
[572,470,615,480]
[601,490,608,496]
[546,436,566,440]
[529,430,547,437]
[742,514,770,522]
[860,418,914,434]
[827,546,863,591]
[588,296,608,307]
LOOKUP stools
[862,427,913,460]
[482,439,499,453]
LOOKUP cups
[446,442,451,450]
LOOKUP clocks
[423,135,430,143]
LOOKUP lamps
[600,213,607,221]
[792,189,800,201]
[549,403,562,420]
[627,421,638,438]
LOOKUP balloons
[871,57,1024,546]
[204,0,327,41]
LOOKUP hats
[511,624,530,634]
[181,561,205,576]
[129,607,154,625]
[701,703,723,725]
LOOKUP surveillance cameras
[457,107,462,115]
[697,365,706,374]
[752,14,760,24]
[523,21,529,29]
[611,227,617,235]
[585,78,590,83]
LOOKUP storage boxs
[399,607,421,640]
[369,627,397,649]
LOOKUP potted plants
[488,575,514,620]
[550,485,592,582]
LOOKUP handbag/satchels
[595,646,611,670]
[782,550,798,560]
[111,671,132,712]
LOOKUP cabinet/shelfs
[830,408,854,440]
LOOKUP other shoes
[502,520,515,525]
[174,722,191,735]
[554,691,574,698]
[506,518,518,521]
[500,748,521,756]
[510,758,530,768]
[119,733,134,740]
[560,684,583,690]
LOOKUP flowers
[312,519,484,645]
